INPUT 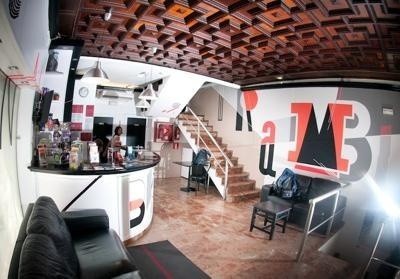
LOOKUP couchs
[260,174,347,235]
[9,196,142,279]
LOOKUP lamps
[80,6,112,86]
[136,46,159,108]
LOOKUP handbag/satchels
[193,149,206,165]
[275,169,297,199]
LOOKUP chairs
[190,149,211,194]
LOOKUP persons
[94,136,104,162]
[98,133,109,163]
[102,126,126,163]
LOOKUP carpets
[125,240,212,279]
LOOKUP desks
[173,161,209,191]
